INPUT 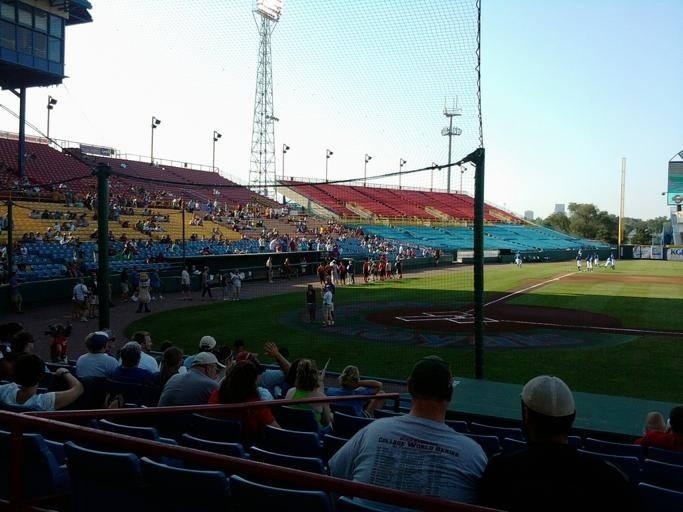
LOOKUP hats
[84,328,113,346]
[192,352,227,370]
[199,335,216,350]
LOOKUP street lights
[282,144,468,195]
[149,115,161,167]
[45,94,58,139]
[211,130,222,175]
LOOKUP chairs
[0,138,317,285]
[316,183,609,256]
[646,446,683,464]
[446,420,470,435]
[334,412,374,434]
[472,422,522,437]
[280,405,321,430]
[2,381,49,395]
[193,412,247,445]
[569,434,581,446]
[251,446,325,475]
[575,449,639,487]
[0,431,75,512]
[140,456,229,512]
[586,438,641,460]
[46,361,77,374]
[99,418,161,441]
[643,458,683,491]
[336,495,400,512]
[324,432,348,450]
[230,475,330,512]
[464,434,500,455]
[182,432,250,459]
[638,481,683,512]
[265,424,322,456]
[63,440,142,512]
[372,408,404,418]
[504,437,528,453]
[191,412,242,443]
[472,422,522,444]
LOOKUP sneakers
[519,375,575,417]
[80,318,88,322]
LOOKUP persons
[324,354,487,510]
[634,411,665,445]
[2,152,615,438]
[475,374,633,512]
[641,406,682,453]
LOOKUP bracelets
[61,371,70,378]
